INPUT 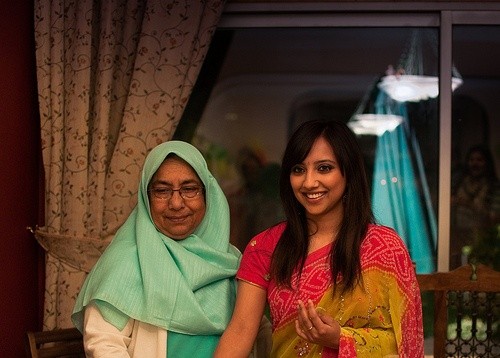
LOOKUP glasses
[149,179,202,199]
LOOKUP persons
[212,118,425,358]
[71,140,271,358]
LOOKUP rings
[307,326,313,331]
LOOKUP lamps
[348,28,465,137]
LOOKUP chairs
[27,327,87,357]
